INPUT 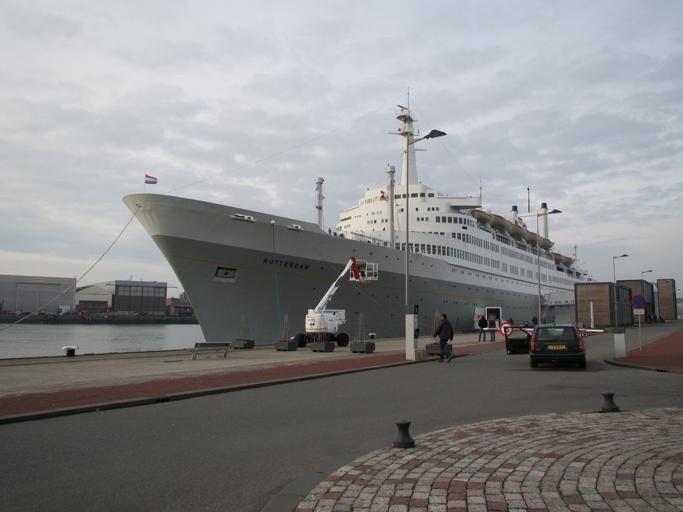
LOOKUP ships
[121,85,597,350]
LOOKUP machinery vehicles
[282,253,357,357]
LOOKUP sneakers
[439,359,443,362]
[448,356,452,361]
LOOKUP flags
[143,174,157,185]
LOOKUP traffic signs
[499,321,512,336]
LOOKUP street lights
[532,206,561,325]
[612,253,628,327]
[400,125,448,361]
[639,268,652,296]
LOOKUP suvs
[501,320,589,371]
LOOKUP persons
[530,316,537,326]
[433,313,454,364]
[488,318,499,342]
[476,316,487,342]
[507,317,513,324]
